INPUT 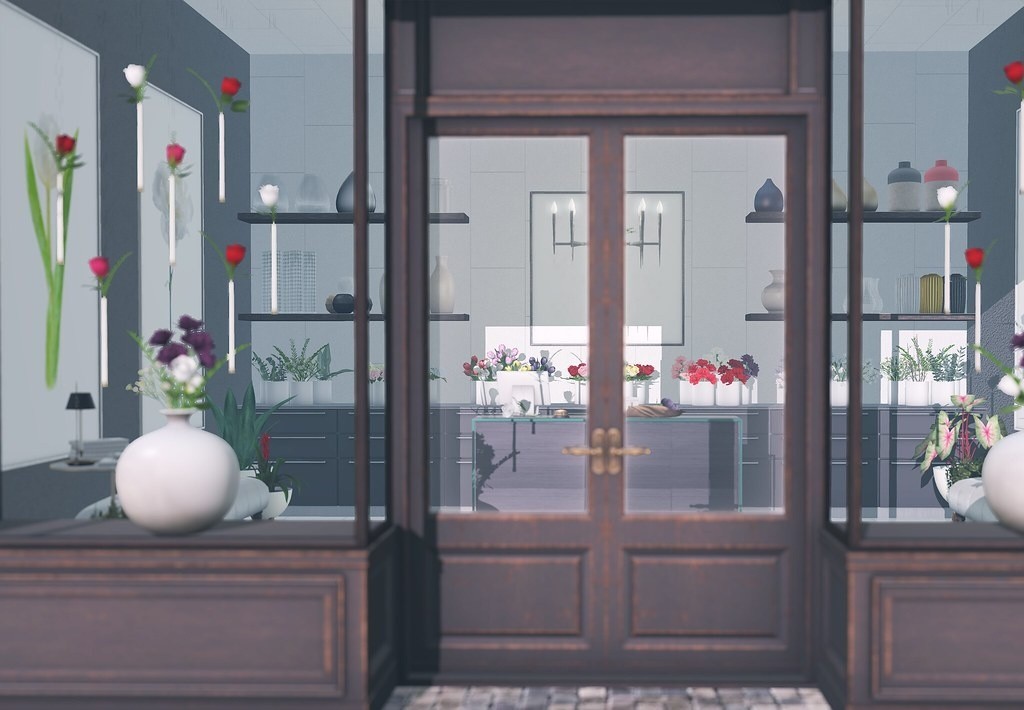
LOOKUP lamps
[67,392,97,467]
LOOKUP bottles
[754,178,782,213]
[863,178,878,210]
[887,159,959,211]
[336,170,376,212]
[787,315,789,317]
[832,178,846,211]
[761,270,785,314]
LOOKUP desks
[49,460,116,504]
[470,414,744,512]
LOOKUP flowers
[20,119,89,389]
[163,143,196,179]
[963,237,1000,283]
[933,178,972,223]
[249,183,281,222]
[119,49,161,106]
[254,431,302,503]
[197,227,246,282]
[462,343,655,382]
[991,61,1024,101]
[369,362,385,384]
[186,66,251,114]
[670,346,760,385]
[967,321,1024,415]
[125,315,252,409]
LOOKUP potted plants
[910,395,1007,503]
[775,337,967,406]
[261,357,289,404]
[429,367,447,403]
[313,344,356,405]
[271,337,329,405]
[203,377,301,520]
[251,351,270,403]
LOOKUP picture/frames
[529,191,685,346]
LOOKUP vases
[716,381,741,407]
[690,382,714,406]
[740,382,750,404]
[371,382,384,406]
[981,428,1024,531]
[246,487,294,520]
[634,381,646,404]
[679,380,692,405]
[115,406,241,536]
[624,380,634,401]
[468,376,585,406]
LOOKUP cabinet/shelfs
[745,211,982,321]
[235,212,472,323]
[234,402,988,508]
